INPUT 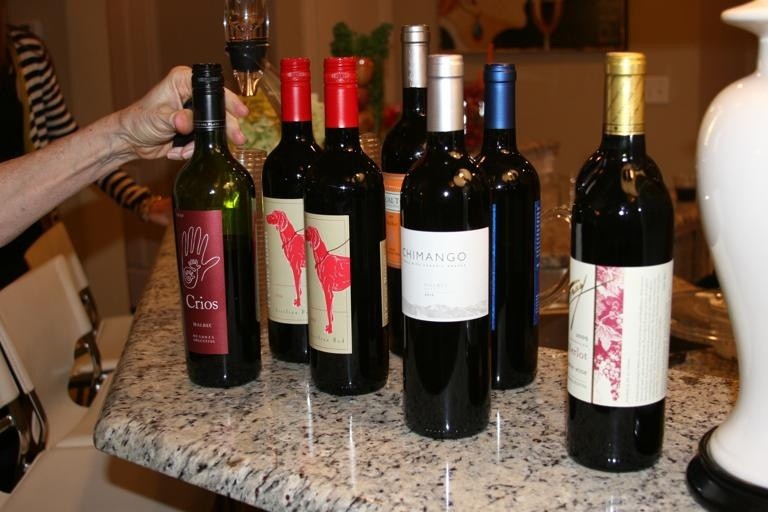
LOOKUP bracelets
[137,192,163,224]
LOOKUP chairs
[23,222,139,401]
[0,352,221,511]
[0,249,121,447]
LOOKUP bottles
[169,63,261,389]
[686,0,768,511]
[475,63,539,393]
[302,55,391,397]
[568,48,674,468]
[382,24,434,360]
[261,58,324,367]
[399,53,492,439]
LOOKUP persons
[0,65,249,246]
[0,20,174,242]
